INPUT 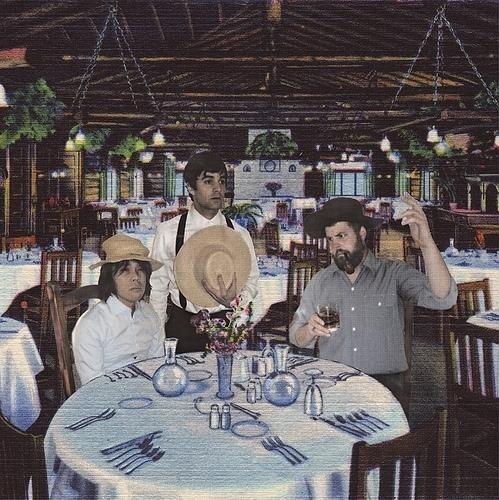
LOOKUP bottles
[241,355,265,379]
[209,402,222,430]
[152,338,188,398]
[222,404,232,430]
[5,238,67,264]
[264,345,298,405]
[442,237,498,263]
[246,378,263,404]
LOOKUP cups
[316,302,343,333]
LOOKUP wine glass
[303,368,326,415]
[258,335,278,357]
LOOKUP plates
[304,376,335,388]
[118,398,154,410]
[227,419,269,438]
[186,369,213,384]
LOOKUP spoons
[332,407,389,437]
[103,369,137,383]
[110,434,167,477]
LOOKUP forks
[64,406,118,432]
[258,434,310,466]
[174,353,207,366]
[330,371,365,382]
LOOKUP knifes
[129,362,154,382]
[319,416,361,440]
[102,431,164,453]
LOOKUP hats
[173,227,252,308]
[304,197,385,239]
[90,235,166,271]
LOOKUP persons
[289,190,458,410]
[68,233,167,391]
[148,149,261,356]
